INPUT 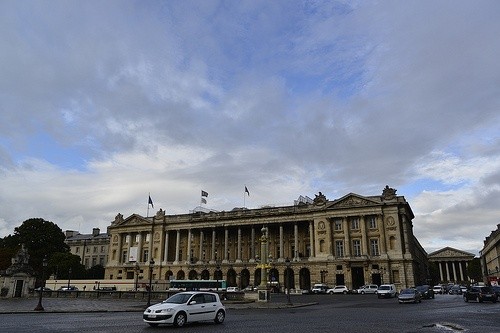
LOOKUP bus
[169,279,226,291]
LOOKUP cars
[377,284,396,299]
[142,291,226,326]
[415,285,434,299]
[312,283,326,294]
[326,285,349,294]
[398,288,423,303]
[433,284,467,295]
[357,284,378,294]
[227,287,239,292]
[463,286,497,303]
[57,286,79,291]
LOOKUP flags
[245,186,249,196]
[202,198,207,204]
[148,196,153,208]
[202,190,208,197]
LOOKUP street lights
[34,253,48,310]
[149,258,155,291]
[135,263,140,290]
